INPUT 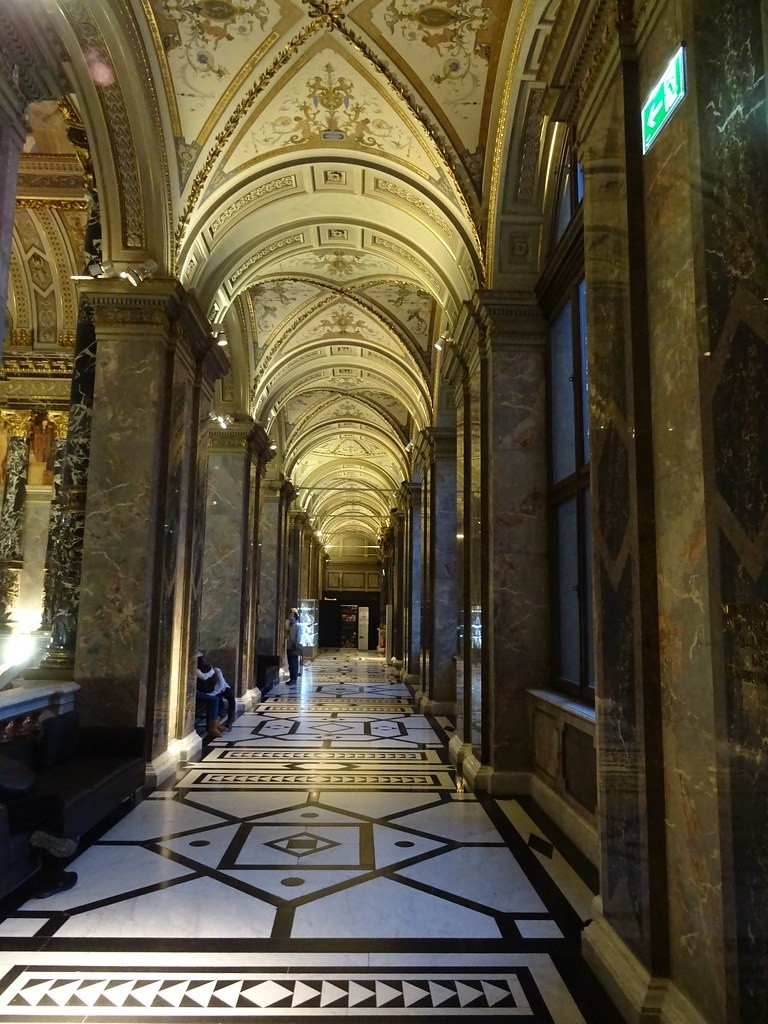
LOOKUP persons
[195,649,235,738]
[285,612,301,686]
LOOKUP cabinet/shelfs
[298,599,319,659]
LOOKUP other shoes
[285,679,296,685]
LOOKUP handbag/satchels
[292,642,303,655]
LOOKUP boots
[216,717,229,732]
[206,719,223,737]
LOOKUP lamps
[434,330,455,350]
[217,331,228,346]
[294,484,302,495]
[88,260,115,276]
[404,444,412,453]
[269,435,278,450]
[220,417,235,429]
[125,259,159,287]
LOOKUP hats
[197,651,203,657]
